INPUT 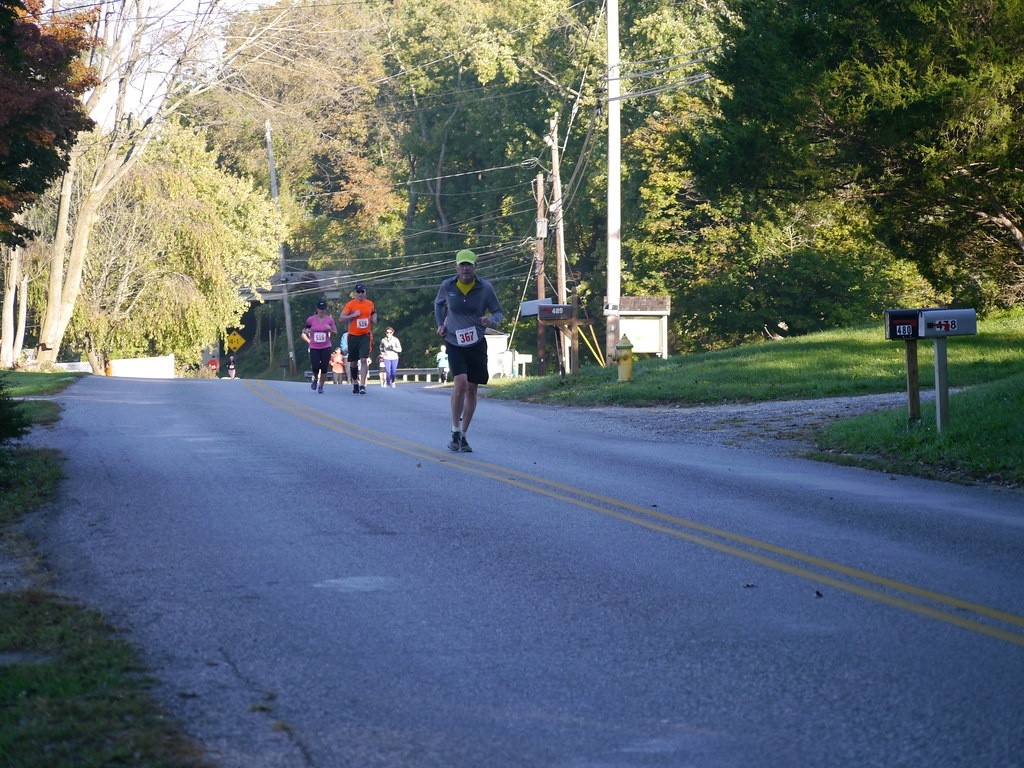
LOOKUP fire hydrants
[607,333,634,384]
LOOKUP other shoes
[318,384,323,393]
[392,382,395,388]
[311,379,317,390]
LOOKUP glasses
[356,290,365,293]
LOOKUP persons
[208,355,220,375]
[379,326,402,389]
[226,355,236,380]
[307,301,337,394]
[300,326,322,384]
[339,284,375,395]
[378,355,387,388]
[434,249,504,452]
[436,345,450,385]
[329,328,372,385]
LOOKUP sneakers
[447,430,461,451]
[353,385,359,394]
[460,438,472,453]
[359,386,365,394]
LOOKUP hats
[355,284,366,290]
[455,250,477,265]
[317,302,327,309]
[230,356,234,359]
